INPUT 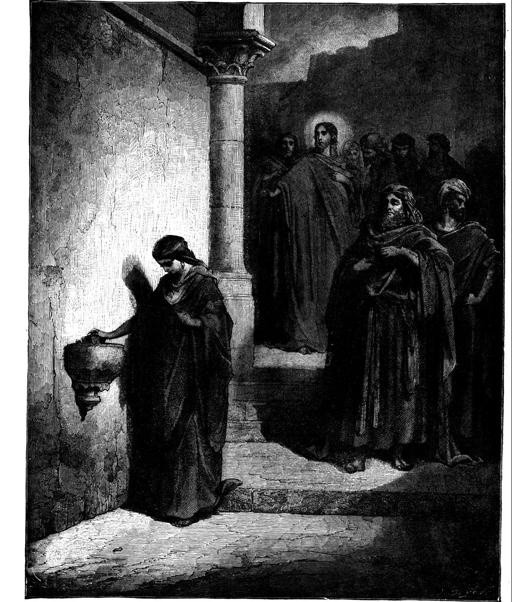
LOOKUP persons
[90,235,243,527]
